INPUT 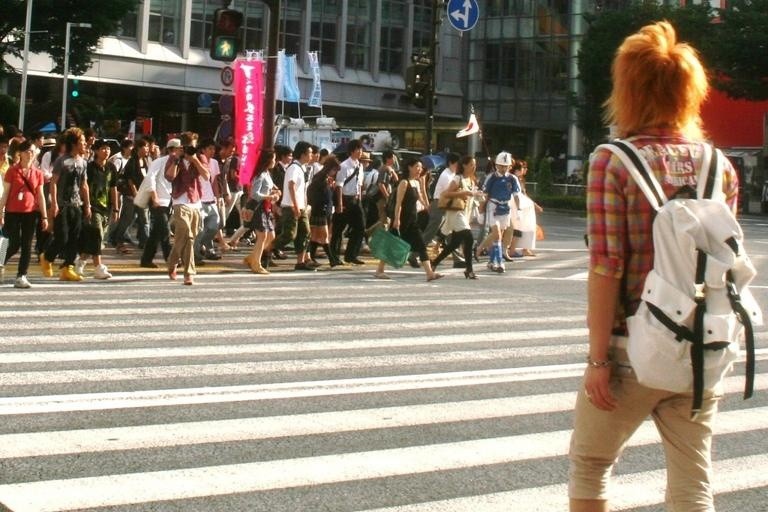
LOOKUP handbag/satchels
[241,200,263,228]
[494,203,511,217]
[366,183,382,205]
[116,173,137,195]
[368,228,410,269]
[437,189,468,210]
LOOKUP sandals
[222,245,241,255]
[242,256,269,275]
[425,272,446,281]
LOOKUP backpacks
[587,138,763,420]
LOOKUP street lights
[59,21,93,130]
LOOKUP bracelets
[112,208,120,212]
[174,158,180,165]
[586,355,614,368]
[83,205,91,208]
[41,216,48,219]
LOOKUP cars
[95,138,125,158]
[393,148,444,198]
[761,180,767,215]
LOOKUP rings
[585,394,592,401]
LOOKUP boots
[58,266,85,282]
[39,253,53,277]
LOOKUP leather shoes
[193,252,222,265]
[168,263,194,285]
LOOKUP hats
[95,139,113,149]
[360,151,374,163]
[40,137,57,149]
[166,138,181,149]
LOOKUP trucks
[271,116,400,176]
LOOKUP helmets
[495,151,513,167]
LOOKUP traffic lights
[210,10,241,61]
[407,50,432,106]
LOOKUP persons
[133,138,183,268]
[39,129,91,281]
[407,152,485,279]
[373,158,445,282]
[261,141,342,272]
[107,137,160,254]
[193,136,281,274]
[473,151,542,273]
[164,131,211,285]
[2,141,48,287]
[330,140,400,265]
[566,19,739,512]
[2,129,95,270]
[73,138,120,280]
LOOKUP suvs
[724,154,749,213]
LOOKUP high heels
[464,269,478,279]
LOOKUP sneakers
[487,262,505,273]
[74,259,89,273]
[12,275,32,289]
[504,248,535,261]
[93,266,113,280]
[270,249,365,271]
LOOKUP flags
[455,114,480,139]
